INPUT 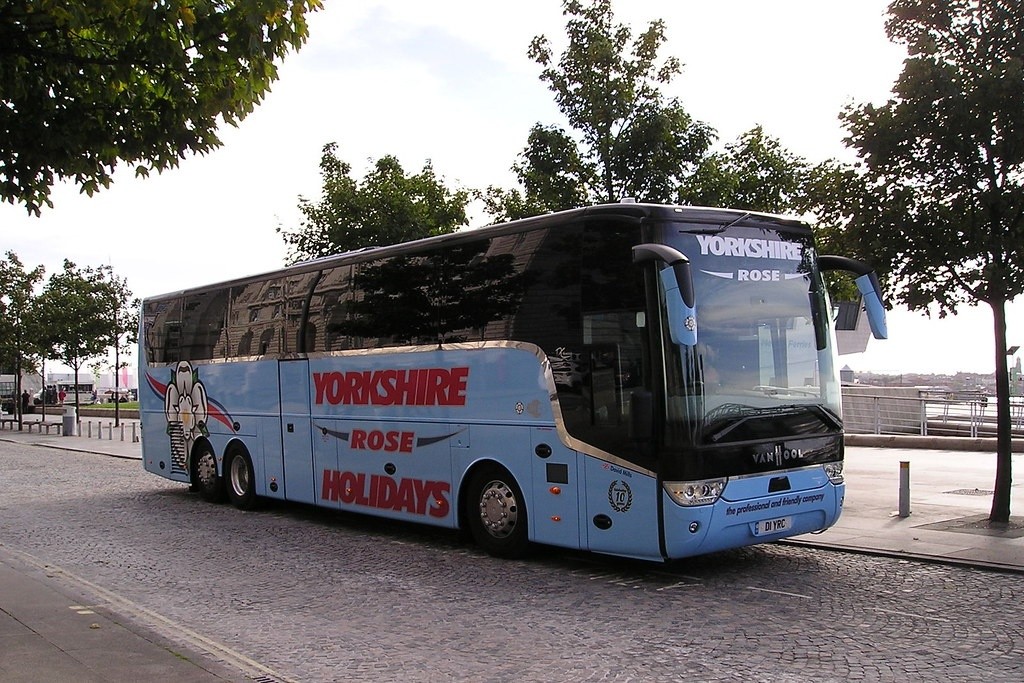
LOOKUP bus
[32,381,95,406]
[136,196,888,572]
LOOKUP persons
[91,390,98,405]
[21,390,31,414]
[58,387,66,404]
[40,384,57,405]
[126,390,134,403]
